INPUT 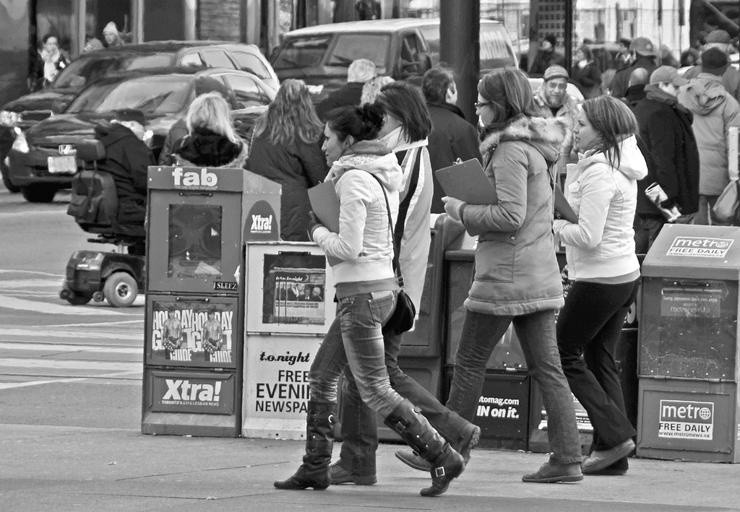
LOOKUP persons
[103,22,124,47]
[203,312,224,360]
[94,110,157,226]
[163,310,184,359]
[31,32,69,92]
[393,65,582,483]
[277,283,323,301]
[329,83,482,485]
[274,100,464,496]
[553,94,649,474]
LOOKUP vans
[268,17,519,104]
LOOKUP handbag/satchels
[383,290,416,335]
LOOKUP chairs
[69,136,148,256]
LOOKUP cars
[511,37,529,73]
[8,68,279,204]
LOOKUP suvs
[1,39,282,194]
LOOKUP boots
[275,401,337,489]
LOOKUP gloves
[661,197,677,208]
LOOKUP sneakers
[383,398,481,496]
[581,439,636,476]
[328,464,377,485]
[523,459,584,483]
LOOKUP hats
[629,38,657,58]
[649,64,689,86]
[702,48,729,74]
[114,110,149,126]
[544,65,569,80]
